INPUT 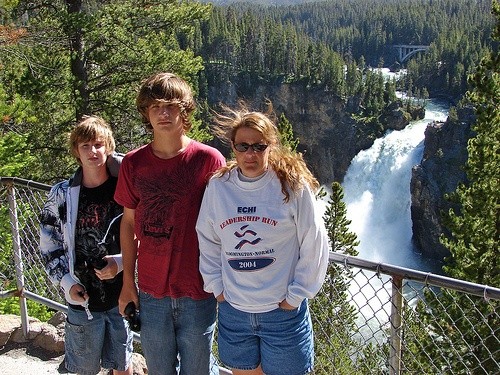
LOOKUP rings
[77,290,86,297]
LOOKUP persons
[113,71,227,375]
[194,111,330,375]
[38,114,142,375]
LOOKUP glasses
[233,143,268,152]
